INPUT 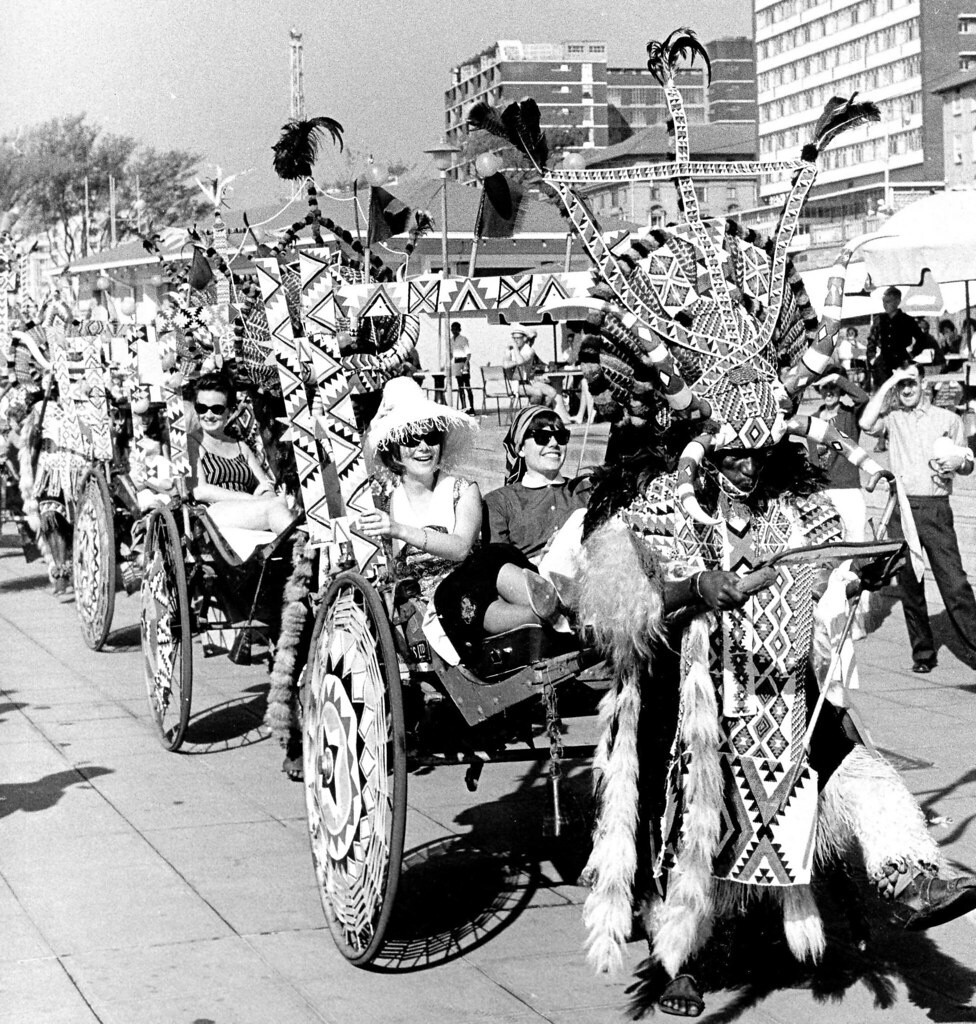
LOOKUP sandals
[288,741,306,781]
[659,973,705,1017]
[888,875,976,932]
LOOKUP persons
[0,287,976,1024]
[858,358,976,673]
[579,447,976,1024]
[439,320,478,417]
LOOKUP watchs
[960,460,969,473]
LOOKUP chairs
[426,362,462,409]
[480,365,526,425]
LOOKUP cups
[578,365,581,370]
[564,366,575,370]
[551,366,556,373]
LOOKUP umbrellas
[861,192,976,370]
[488,277,593,372]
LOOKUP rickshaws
[75,364,915,966]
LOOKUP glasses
[512,336,524,339]
[194,401,228,415]
[524,429,571,445]
[395,429,443,448]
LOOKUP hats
[508,322,528,337]
[361,376,480,481]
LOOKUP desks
[536,370,585,424]
[414,372,445,398]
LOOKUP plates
[563,370,582,371]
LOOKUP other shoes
[465,409,476,415]
[568,416,583,424]
[589,410,597,424]
[912,662,933,673]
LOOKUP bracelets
[421,529,428,552]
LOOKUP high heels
[549,571,576,612]
[523,568,575,635]
